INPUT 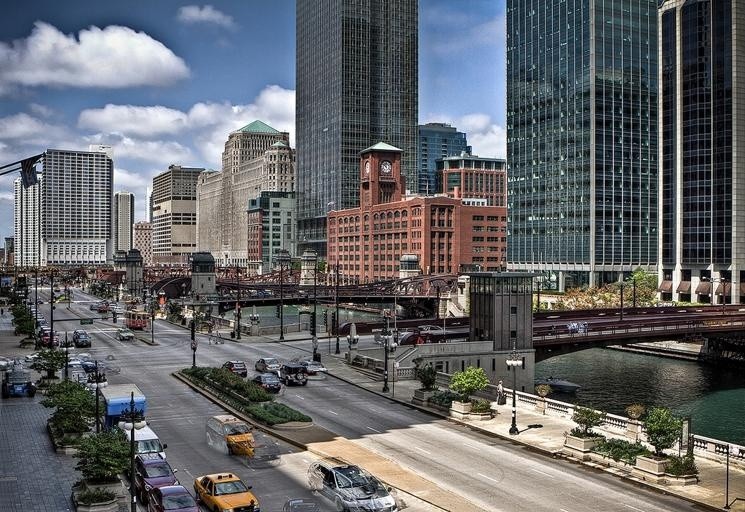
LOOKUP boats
[535,377,581,395]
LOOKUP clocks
[365,161,369,173]
[380,161,392,173]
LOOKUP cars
[221,356,280,393]
[388,324,445,344]
[48,296,55,303]
[135,452,205,512]
[205,414,257,456]
[306,361,327,375]
[29,304,60,346]
[2,365,38,398]
[115,326,135,341]
[89,299,124,316]
[127,295,143,306]
[0,352,104,368]
[305,456,400,512]
[193,472,260,512]
[72,329,92,348]
[36,296,45,306]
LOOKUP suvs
[280,363,308,385]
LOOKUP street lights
[271,250,291,340]
[29,265,41,330]
[532,268,546,312]
[616,281,627,321]
[630,275,641,308]
[43,267,61,349]
[82,359,107,433]
[702,273,719,305]
[506,341,524,435]
[118,391,146,512]
[718,277,729,314]
[380,307,392,396]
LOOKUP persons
[496,379,505,405]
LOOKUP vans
[123,424,167,452]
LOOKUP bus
[122,307,155,331]
